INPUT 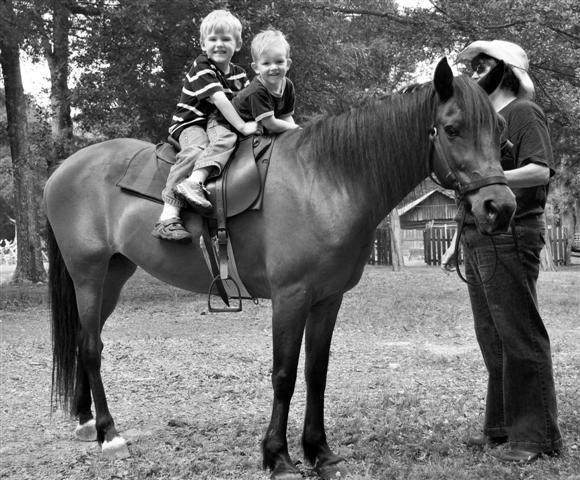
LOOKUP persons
[150,10,264,242]
[441,39,562,466]
[172,30,303,214]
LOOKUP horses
[39,57,521,480]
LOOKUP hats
[457,39,536,101]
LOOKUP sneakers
[171,179,213,214]
[151,216,192,241]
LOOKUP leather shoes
[497,447,539,464]
[462,433,503,446]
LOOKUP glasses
[470,62,488,75]
